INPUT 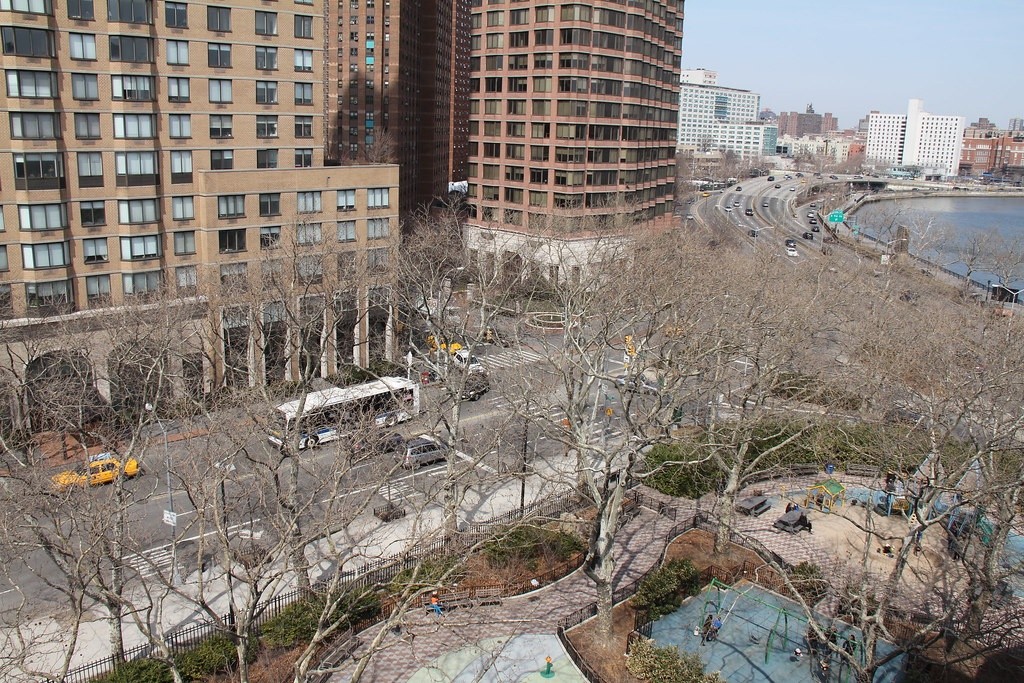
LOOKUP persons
[431,592,445,618]
[701,615,722,645]
[786,502,799,512]
[800,512,813,534]
[806,624,857,683]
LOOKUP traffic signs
[828,208,844,222]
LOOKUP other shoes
[706,638,711,642]
[811,529,814,534]
[701,642,705,646]
[441,613,445,618]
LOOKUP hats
[794,504,797,506]
[795,648,801,653]
[885,543,890,546]
[433,590,437,595]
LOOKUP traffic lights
[628,347,635,355]
[625,336,633,345]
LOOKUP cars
[736,186,742,191]
[785,247,798,257]
[616,374,660,396]
[823,237,832,242]
[854,173,914,179]
[477,328,513,348]
[790,186,795,191]
[744,207,753,216]
[811,208,817,215]
[795,172,838,180]
[783,174,788,178]
[810,202,816,206]
[809,218,817,224]
[734,200,740,207]
[785,239,796,247]
[762,201,768,207]
[456,372,490,400]
[747,228,758,237]
[803,231,813,240]
[767,175,775,181]
[725,205,732,212]
[900,289,922,305]
[50,452,139,494]
[807,210,814,217]
[810,225,819,232]
[345,429,406,458]
[426,334,462,355]
[786,175,792,180]
[775,182,781,188]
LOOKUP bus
[267,374,420,452]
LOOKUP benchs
[476,588,502,606]
[774,514,786,530]
[734,497,749,510]
[794,520,811,534]
[617,498,642,529]
[754,504,771,518]
[847,463,884,479]
[421,588,472,616]
[742,463,818,483]
[292,628,361,683]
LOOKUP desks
[739,496,767,516]
[778,510,806,534]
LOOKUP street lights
[738,225,775,252]
[715,204,742,225]
[436,266,463,379]
[986,280,990,302]
[991,283,1024,372]
[885,238,907,275]
[962,169,967,183]
[144,402,182,589]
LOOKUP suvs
[393,435,449,470]
[453,349,486,374]
